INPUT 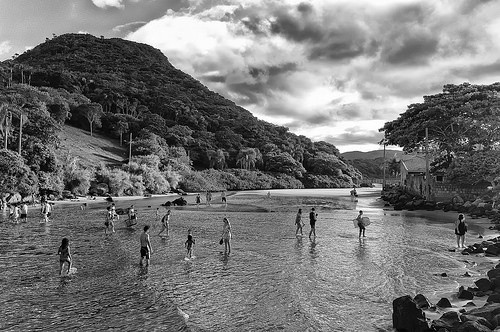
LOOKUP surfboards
[353,216,371,228]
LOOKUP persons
[206,190,212,206]
[309,207,318,239]
[104,207,116,235]
[454,213,468,248]
[57,237,72,275]
[295,209,304,234]
[195,194,201,211]
[109,203,120,221]
[129,205,136,228]
[221,217,232,254]
[184,233,195,258]
[40,192,54,221]
[155,208,160,220]
[157,210,172,236]
[8,202,29,224]
[221,191,227,203]
[139,225,153,267]
[357,210,366,238]
